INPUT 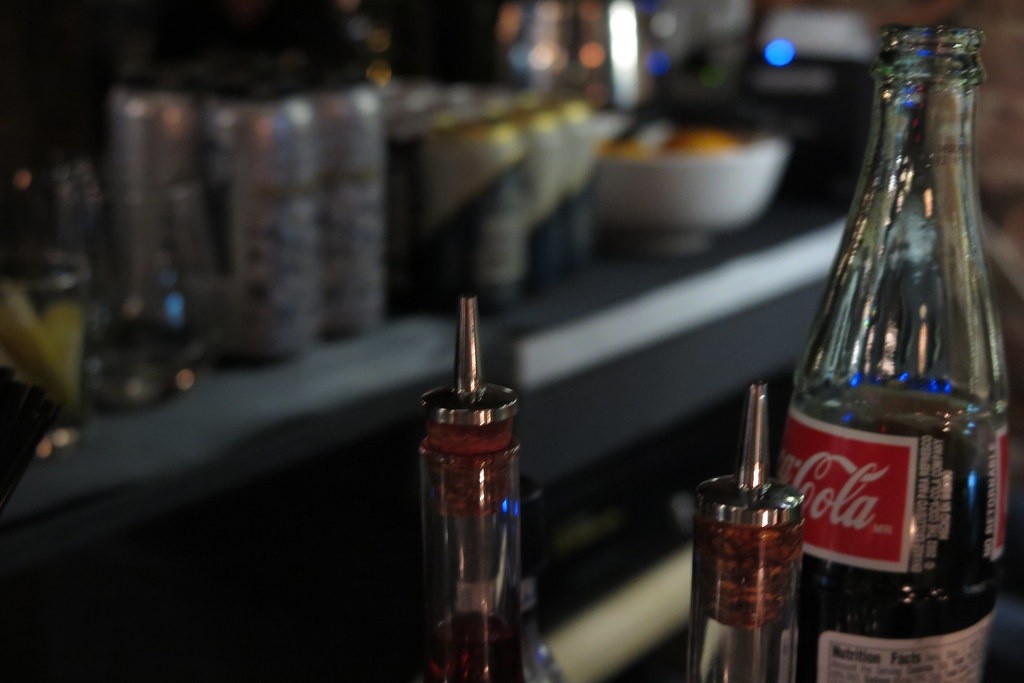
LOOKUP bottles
[416,293,523,683]
[777,20,1014,683]
[679,376,806,683]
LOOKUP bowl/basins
[597,140,789,254]
